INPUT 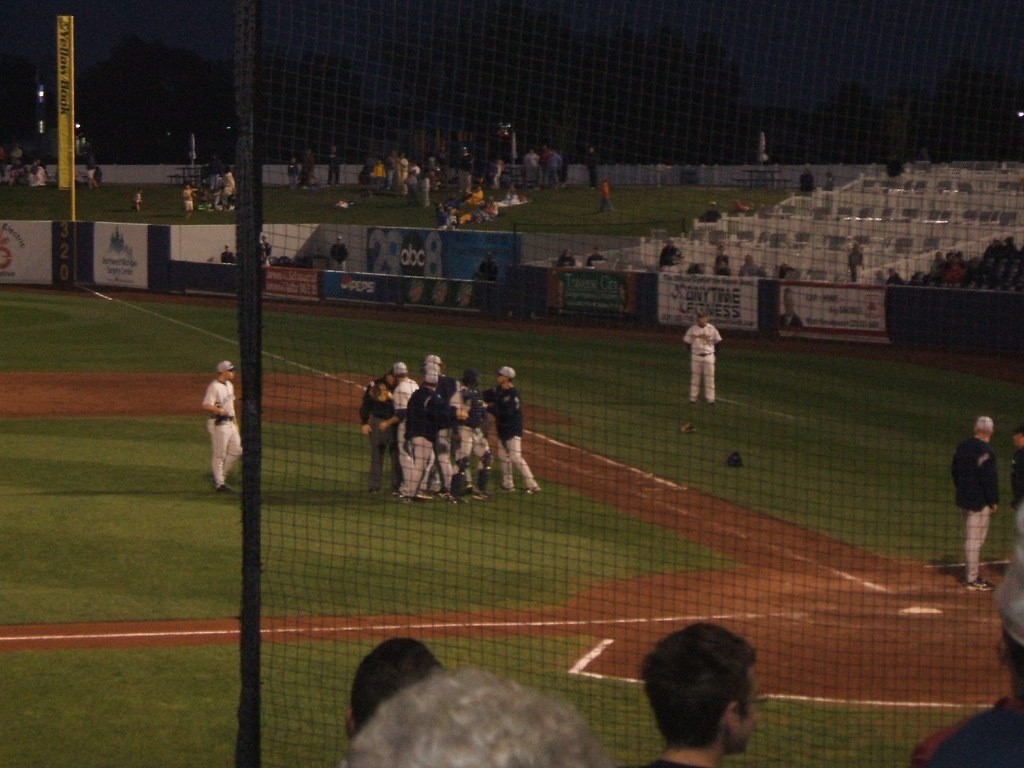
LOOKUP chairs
[575,162,1024,286]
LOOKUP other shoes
[369,483,540,503]
[216,484,235,494]
[706,402,719,408]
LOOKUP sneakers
[966,576,995,592]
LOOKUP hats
[698,312,709,317]
[665,236,674,243]
[425,355,443,365]
[495,366,518,379]
[1013,424,1024,433]
[424,363,442,383]
[393,362,409,374]
[975,416,997,433]
[215,360,238,372]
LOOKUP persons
[950,415,1001,591]
[222,235,349,271]
[0,145,103,190]
[201,360,243,493]
[183,151,236,215]
[359,143,620,230]
[1010,421,1024,510]
[660,236,683,273]
[688,163,1023,293]
[910,505,1023,768]
[557,248,575,266]
[357,355,541,504]
[682,312,722,404]
[641,623,756,768]
[474,251,499,284]
[287,145,339,187]
[347,637,601,768]
[586,246,608,265]
[134,187,143,212]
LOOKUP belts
[210,414,234,420]
[694,353,712,357]
